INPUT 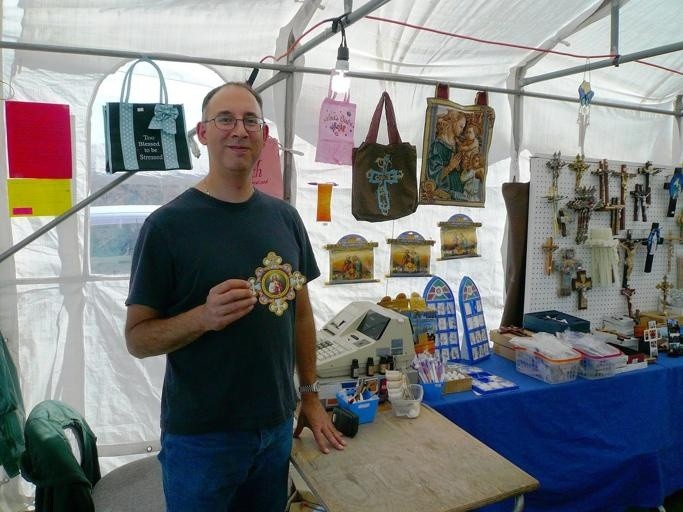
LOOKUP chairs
[19,399,167,512]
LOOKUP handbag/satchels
[103,58,192,173]
[315,68,355,165]
[251,137,283,199]
[351,91,417,222]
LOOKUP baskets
[571,348,621,380]
[511,346,583,385]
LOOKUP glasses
[202,115,265,132]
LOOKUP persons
[410,249,420,273]
[400,249,411,272]
[347,263,355,276]
[454,112,486,171]
[352,255,362,279]
[459,232,469,254]
[460,152,485,202]
[451,230,461,256]
[124,83,350,511]
[268,273,284,295]
[425,109,471,201]
[343,255,352,276]
[579,165,682,286]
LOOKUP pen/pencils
[413,358,444,383]
[307,181,339,186]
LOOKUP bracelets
[298,380,319,395]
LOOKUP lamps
[330,45,352,95]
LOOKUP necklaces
[200,181,255,196]
